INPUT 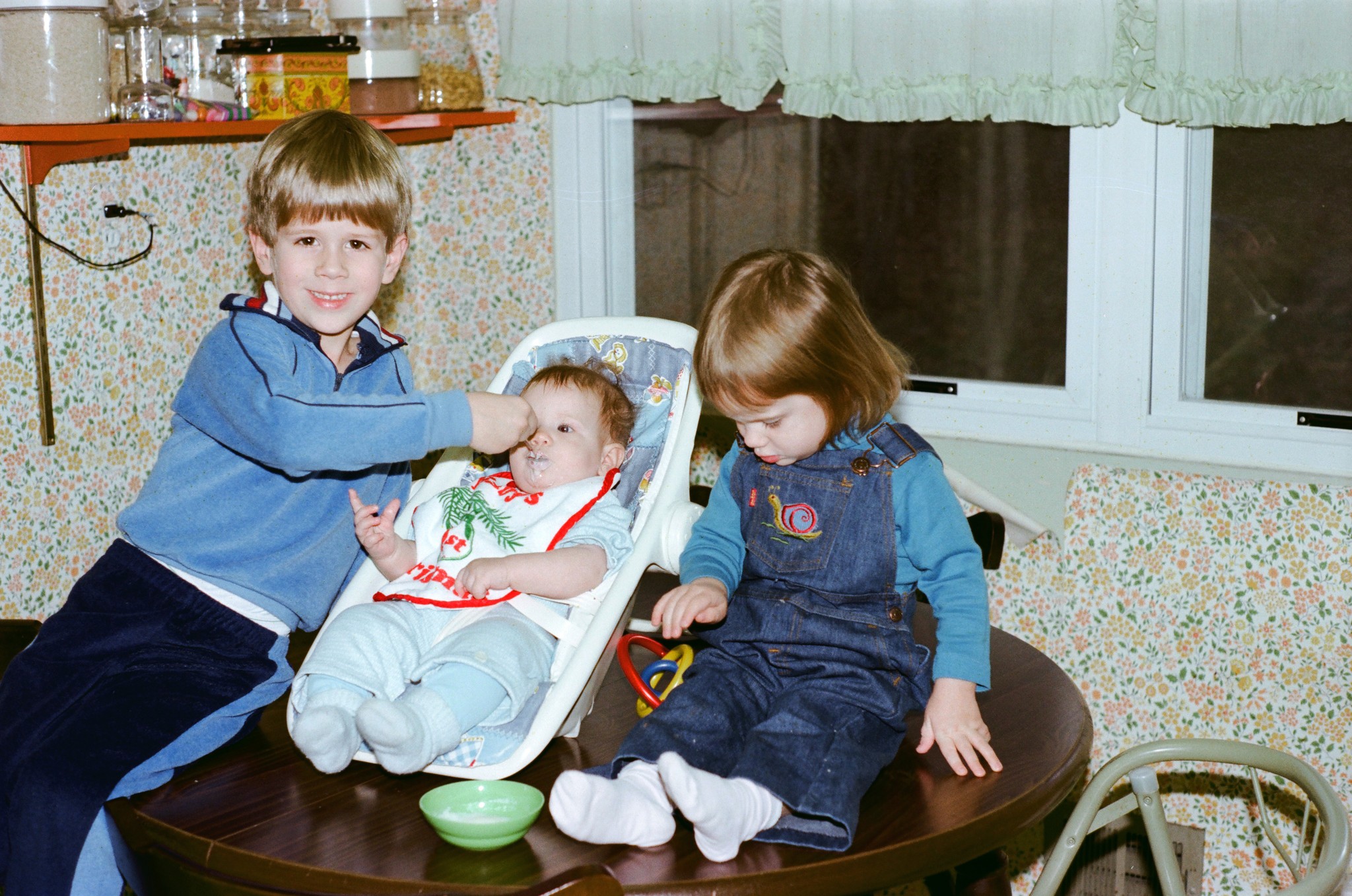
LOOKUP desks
[110,573,1094,896]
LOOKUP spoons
[524,439,547,461]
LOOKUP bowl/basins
[419,780,545,852]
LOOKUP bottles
[109,0,270,122]
[406,0,485,112]
[327,0,420,114]
[0,0,110,125]
[270,8,321,37]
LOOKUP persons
[0,108,540,896]
[291,364,636,775]
[549,248,1003,863]
